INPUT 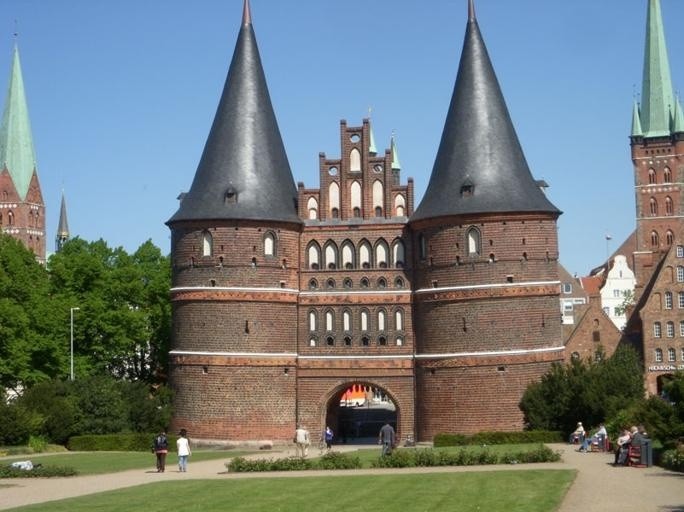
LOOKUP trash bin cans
[598,434,605,452]
[641,438,653,467]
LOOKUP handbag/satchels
[293,437,297,443]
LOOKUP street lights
[70,306,81,381]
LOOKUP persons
[378,419,395,455]
[325,426,333,451]
[153,431,169,472]
[569,422,585,442]
[175,428,192,472]
[294,422,311,458]
[612,425,648,466]
[574,424,607,452]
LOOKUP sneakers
[157,466,165,472]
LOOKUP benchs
[625,441,652,468]
[591,438,609,452]
